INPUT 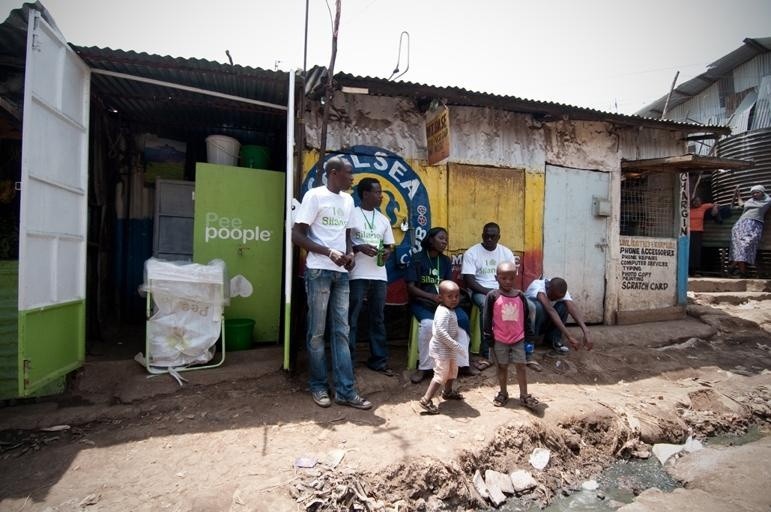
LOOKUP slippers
[525,359,543,372]
[476,358,494,370]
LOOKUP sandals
[494,390,509,406]
[441,389,463,400]
[519,393,540,408]
[419,396,439,414]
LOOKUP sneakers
[410,369,425,383]
[459,366,480,376]
[551,340,569,354]
[312,389,332,407]
[374,364,395,376]
[334,391,372,409]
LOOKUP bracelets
[327,247,334,259]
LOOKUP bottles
[377,239,385,267]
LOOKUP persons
[291,155,374,411]
[417,278,468,416]
[481,260,541,410]
[689,195,718,275]
[404,221,517,384]
[525,275,594,352]
[347,176,397,377]
[725,182,771,278]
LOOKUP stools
[469,303,483,361]
[406,317,423,370]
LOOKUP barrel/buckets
[241,144,272,169]
[204,133,241,167]
[225,318,256,351]
